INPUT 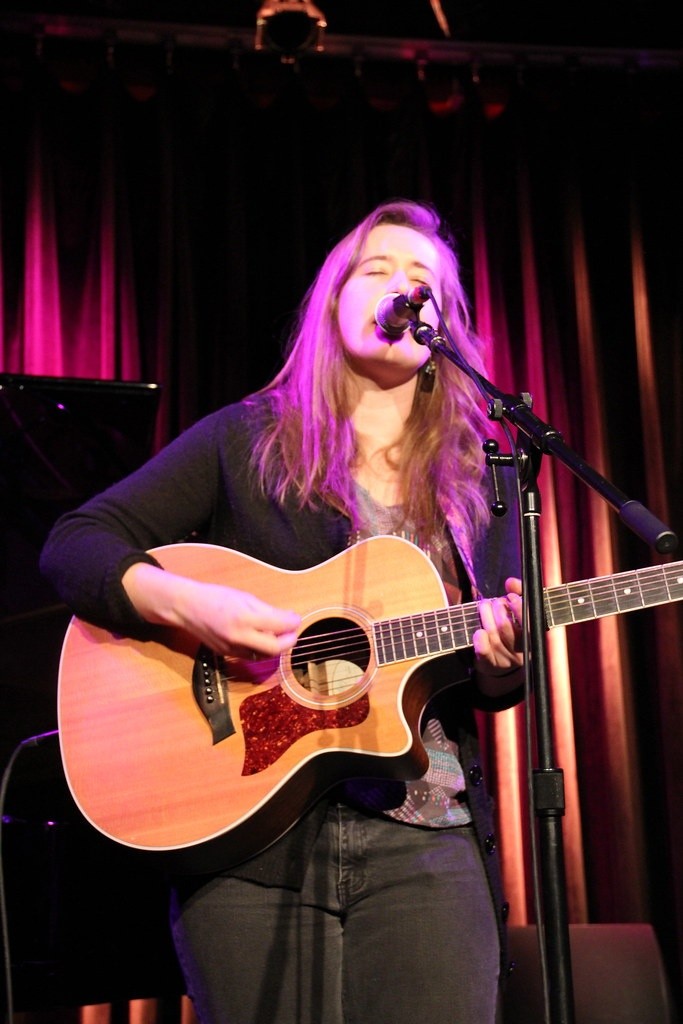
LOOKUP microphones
[375,286,432,335]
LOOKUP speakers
[490,920,671,1024]
[1,373,157,629]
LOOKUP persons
[37,203,532,1024]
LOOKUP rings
[252,653,257,661]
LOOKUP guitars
[56,534,683,876]
